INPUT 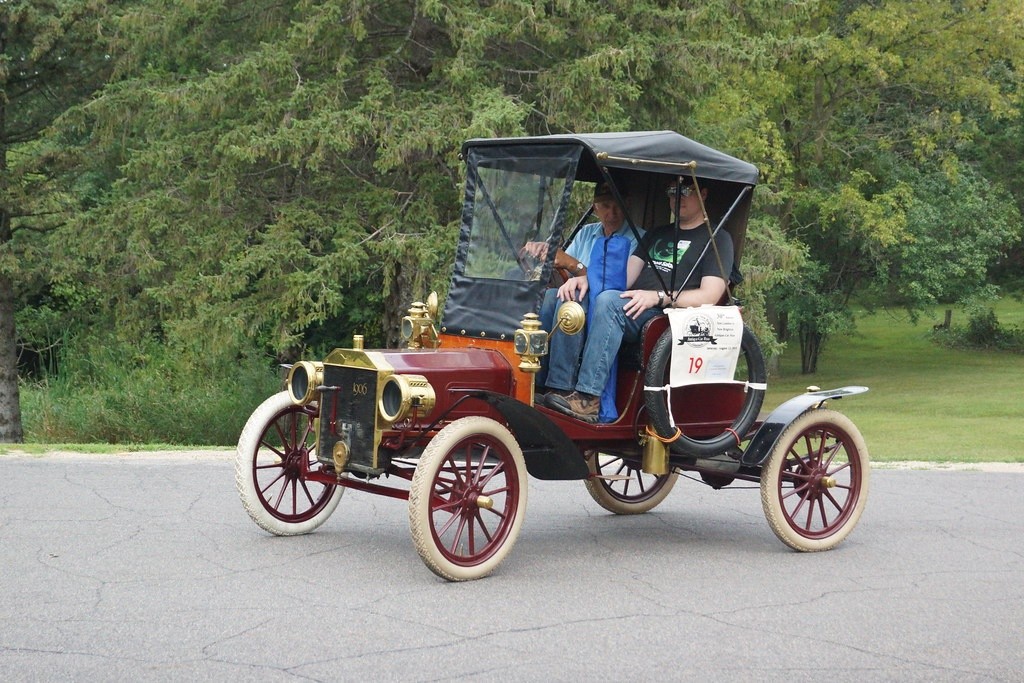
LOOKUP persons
[508,176,647,394]
[534,176,734,423]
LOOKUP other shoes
[544,391,601,423]
[533,388,567,410]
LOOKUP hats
[594,175,627,203]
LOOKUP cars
[234,126,873,583]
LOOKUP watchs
[570,262,584,275]
[654,289,663,308]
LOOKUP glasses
[666,186,699,197]
